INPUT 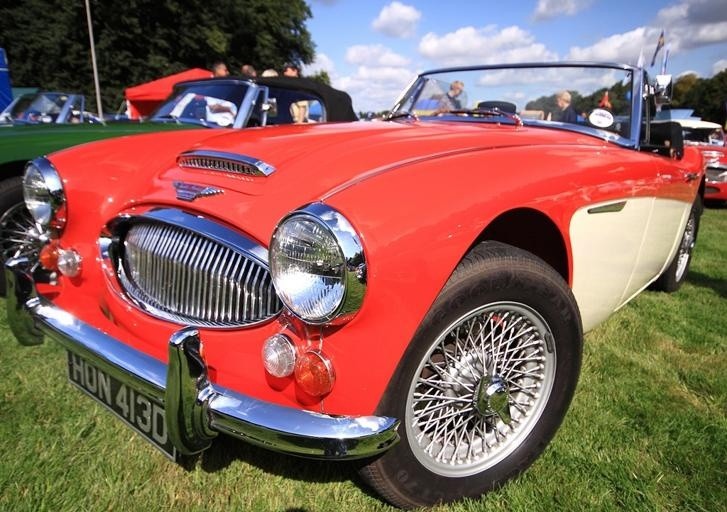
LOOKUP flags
[649,30,665,67]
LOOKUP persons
[203,62,237,129]
[260,67,279,79]
[240,63,257,79]
[437,79,464,115]
[555,90,577,126]
[281,61,309,123]
